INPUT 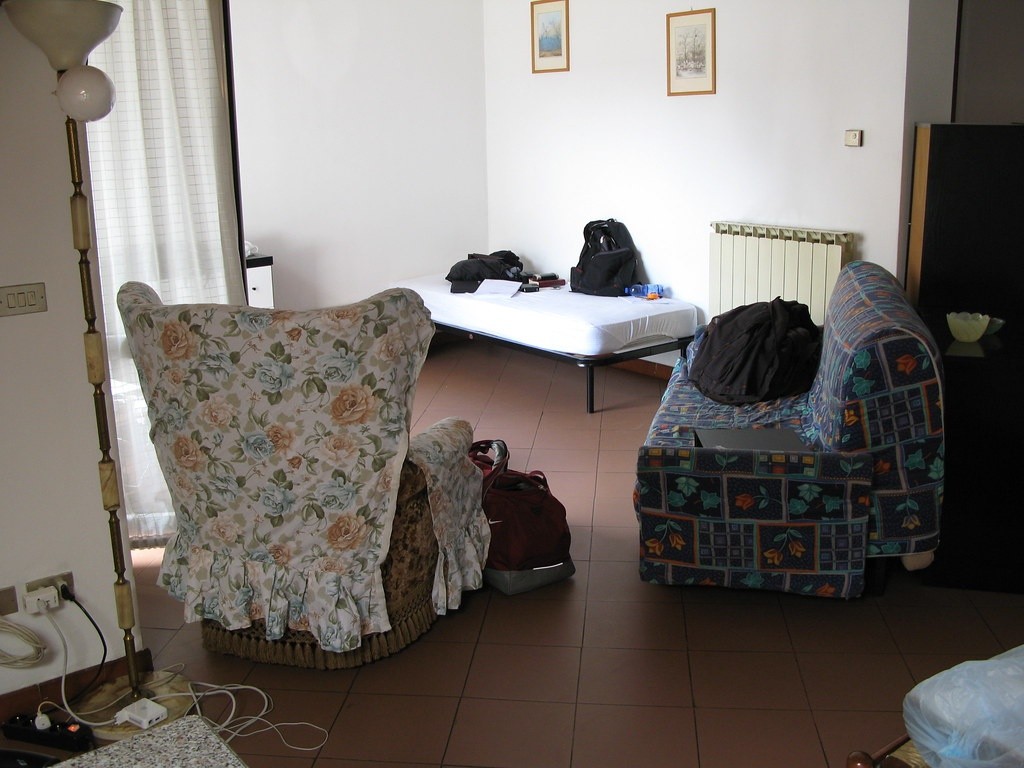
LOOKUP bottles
[624,283,665,297]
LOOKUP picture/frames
[666,9,715,97]
[530,1,569,74]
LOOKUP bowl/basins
[946,311,990,343]
[986,318,1004,335]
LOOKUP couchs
[633,261,945,602]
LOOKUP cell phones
[520,283,539,292]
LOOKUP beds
[394,279,697,414]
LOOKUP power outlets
[24,571,78,608]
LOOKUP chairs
[115,279,491,670]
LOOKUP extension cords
[1,713,93,752]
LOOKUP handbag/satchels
[469,440,576,596]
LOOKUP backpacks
[570,219,639,297]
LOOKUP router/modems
[122,698,168,730]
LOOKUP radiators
[709,218,855,323]
